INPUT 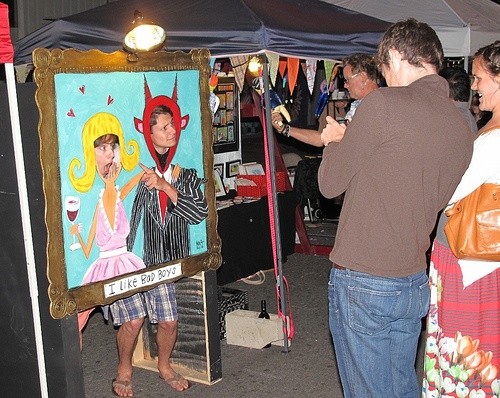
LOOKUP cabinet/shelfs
[327,99,354,122]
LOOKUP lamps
[122,10,167,62]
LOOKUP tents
[1,2,50,398]
[326,0,500,74]
[11,0,394,353]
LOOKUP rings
[274,120,278,125]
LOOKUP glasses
[344,73,361,83]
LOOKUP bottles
[259,300,271,348]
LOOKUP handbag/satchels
[445,182,500,262]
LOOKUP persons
[271,17,472,397]
[421,40,500,398]
[77,106,209,398]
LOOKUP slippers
[159,375,193,391]
[112,378,137,398]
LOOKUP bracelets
[279,123,291,138]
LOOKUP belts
[331,262,346,270]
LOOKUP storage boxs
[237,171,285,199]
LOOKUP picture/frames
[213,77,239,154]
[32,48,222,320]
[214,163,224,178]
[226,160,241,178]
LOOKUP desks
[217,190,297,285]
